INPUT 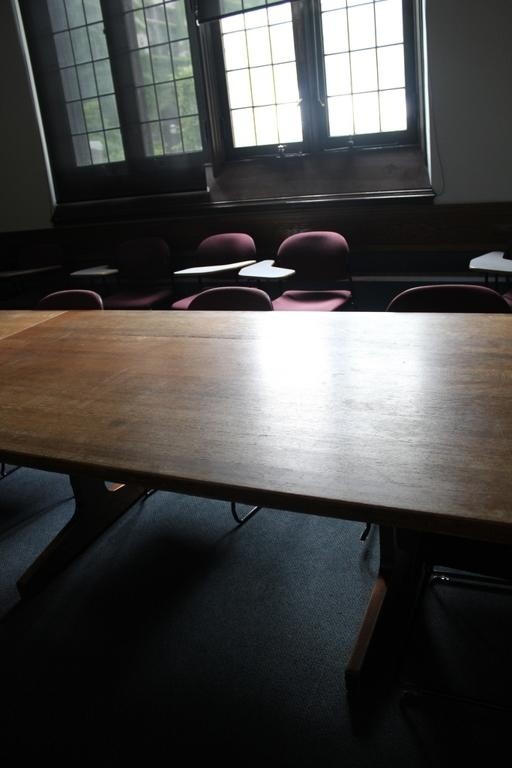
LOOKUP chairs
[37,233,169,308]
[172,232,257,299]
[384,250,511,312]
[237,232,356,293]
[170,284,354,310]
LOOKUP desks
[0,305,512,703]
